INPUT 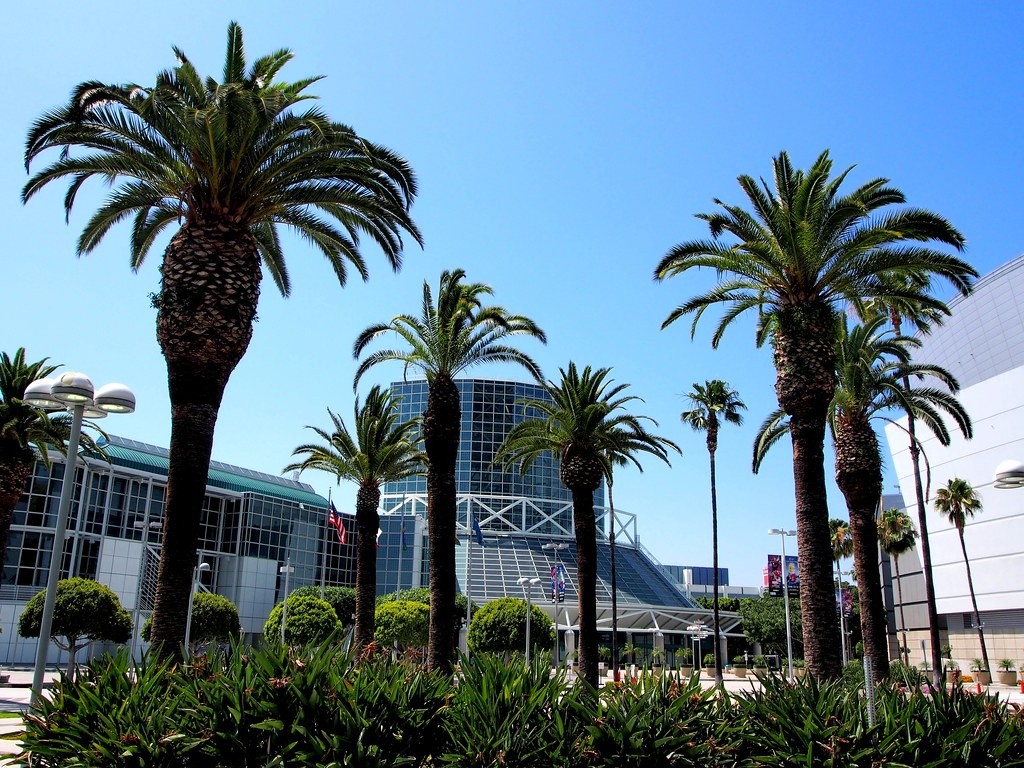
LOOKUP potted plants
[598,644,611,676]
[970,657,991,685]
[733,656,747,677]
[621,641,643,678]
[996,658,1018,685]
[781,657,805,678]
[675,647,695,677]
[752,654,768,676]
[649,647,666,677]
[703,654,716,676]
[941,644,964,682]
[917,661,934,683]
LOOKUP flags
[455,508,488,547]
[328,499,347,545]
[400,498,407,551]
[376,527,383,549]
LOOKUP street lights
[541,543,571,676]
[184,562,211,664]
[767,527,798,685]
[280,558,294,647]
[19,369,137,768]
[129,520,163,680]
[517,577,541,672]
